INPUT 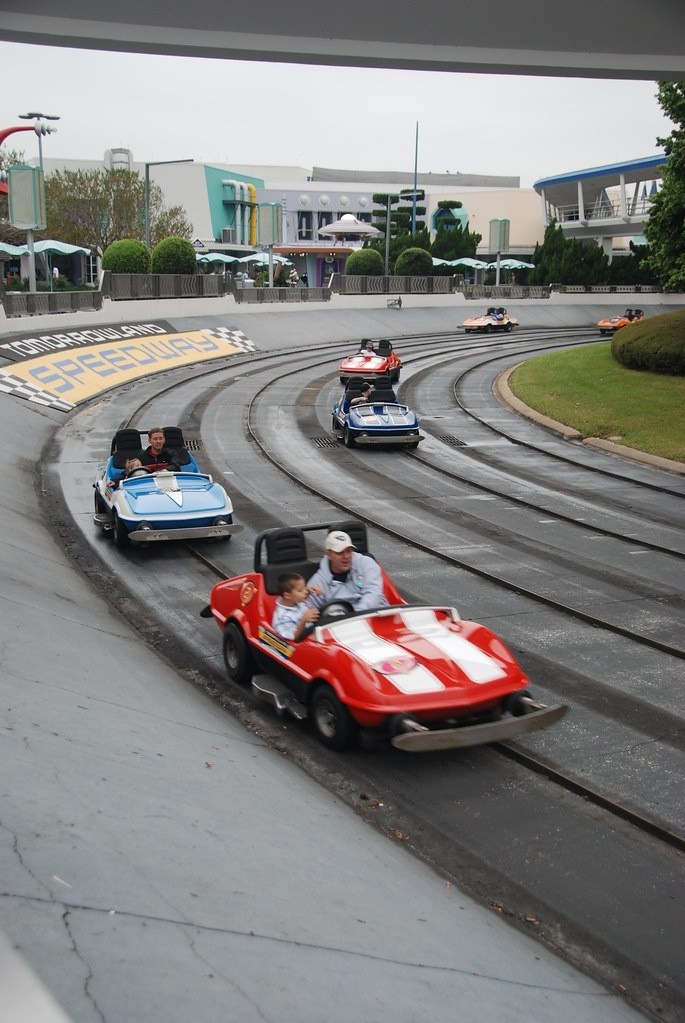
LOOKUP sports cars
[339,338,404,386]
[331,376,425,448]
[461,306,520,334]
[199,519,571,753]
[91,426,246,547]
[596,308,645,334]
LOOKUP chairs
[255,519,376,642]
[111,427,191,472]
[486,307,507,316]
[625,309,641,319]
[359,339,391,356]
[345,376,396,403]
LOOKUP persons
[275,268,307,286]
[510,271,516,285]
[624,311,632,322]
[198,264,207,274]
[242,270,259,287]
[360,341,375,355]
[126,457,142,477]
[106,427,181,487]
[218,264,223,273]
[491,308,504,320]
[351,383,371,405]
[272,572,321,640]
[304,531,390,614]
[53,264,59,285]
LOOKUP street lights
[143,158,196,247]
[384,190,423,276]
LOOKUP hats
[366,341,373,348]
[361,382,374,391]
[325,531,357,553]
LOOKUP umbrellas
[238,253,288,287]
[0,242,31,258]
[253,261,295,266]
[196,253,239,274]
[19,240,91,292]
[431,257,535,284]
